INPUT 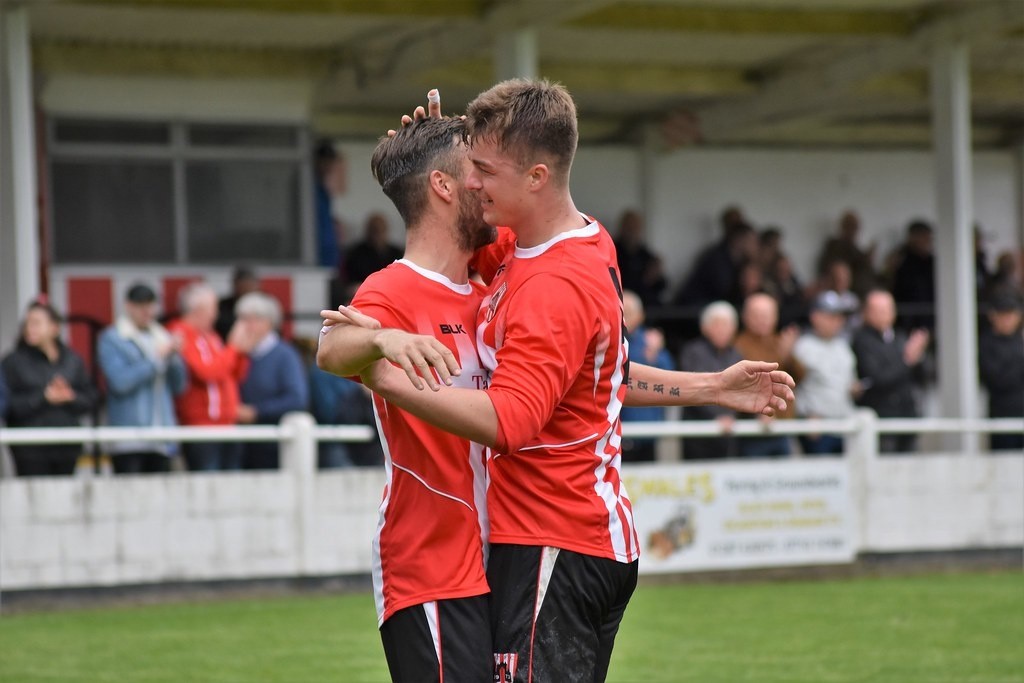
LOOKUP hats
[812,291,843,316]
[126,284,164,316]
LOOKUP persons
[680,206,1024,387]
[96,283,188,473]
[976,287,1023,450]
[232,291,311,469]
[0,302,95,477]
[621,290,674,463]
[792,290,864,456]
[165,281,252,471]
[317,115,796,683]
[610,206,662,300]
[329,216,404,311]
[213,270,257,346]
[312,282,385,465]
[320,78,642,683]
[851,286,934,452]
[678,301,751,458]
[733,294,806,457]
